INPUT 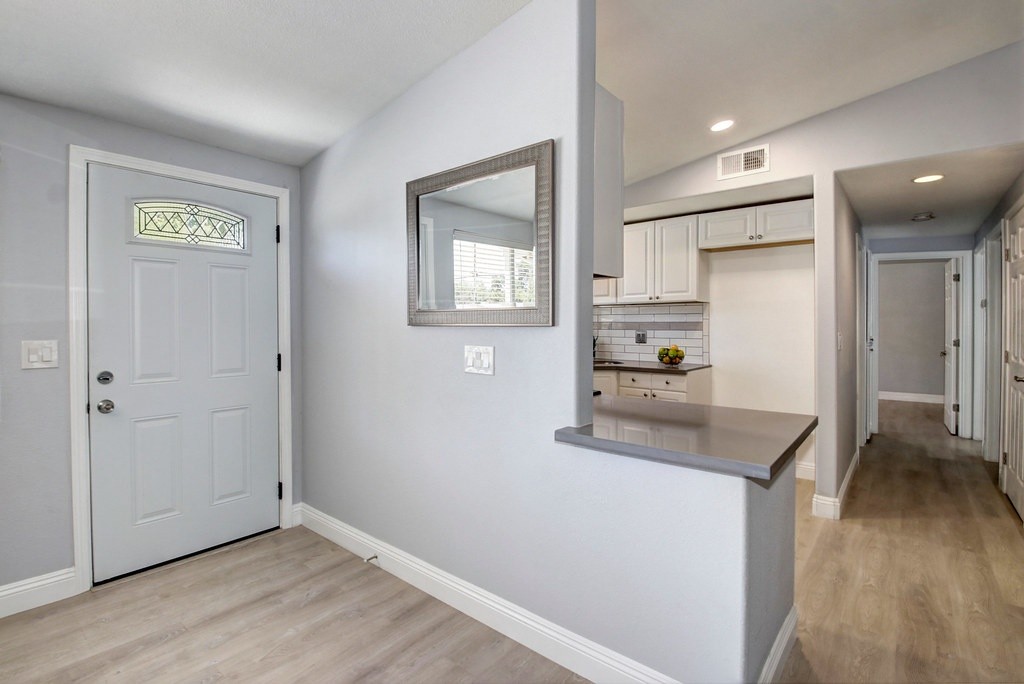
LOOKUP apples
[657,345,684,363]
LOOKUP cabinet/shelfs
[593,278,617,305]
[619,367,712,405]
[592,414,618,442]
[617,214,709,303]
[618,419,711,455]
[698,199,814,249]
[593,371,619,395]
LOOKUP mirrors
[406,139,555,328]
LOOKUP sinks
[593,361,624,365]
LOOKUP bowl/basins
[657,355,685,368]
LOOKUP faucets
[593,336,598,357]
